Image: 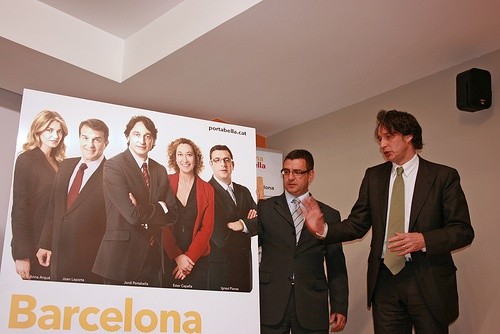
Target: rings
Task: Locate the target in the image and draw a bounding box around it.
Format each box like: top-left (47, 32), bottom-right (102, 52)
top-left (182, 269), bottom-right (187, 274)
top-left (403, 245), bottom-right (406, 249)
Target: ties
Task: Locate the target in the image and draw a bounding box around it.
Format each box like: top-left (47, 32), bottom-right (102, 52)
top-left (291, 198), bottom-right (305, 245)
top-left (65, 163), bottom-right (88, 213)
top-left (227, 185), bottom-right (237, 207)
top-left (142, 163), bottom-right (150, 192)
top-left (383, 167), bottom-right (406, 276)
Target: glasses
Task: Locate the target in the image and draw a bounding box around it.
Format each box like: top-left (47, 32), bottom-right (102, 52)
top-left (213, 158), bottom-right (232, 164)
top-left (281, 169), bottom-right (308, 176)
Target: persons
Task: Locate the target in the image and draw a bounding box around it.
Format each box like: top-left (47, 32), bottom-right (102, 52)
top-left (36, 117), bottom-right (108, 286)
top-left (298, 110), bottom-right (475, 334)
top-left (257, 149), bottom-right (349, 334)
top-left (207, 145), bottom-right (257, 292)
top-left (92, 117), bottom-right (179, 286)
top-left (11, 111), bottom-right (68, 281)
top-left (162, 138), bottom-right (215, 290)
